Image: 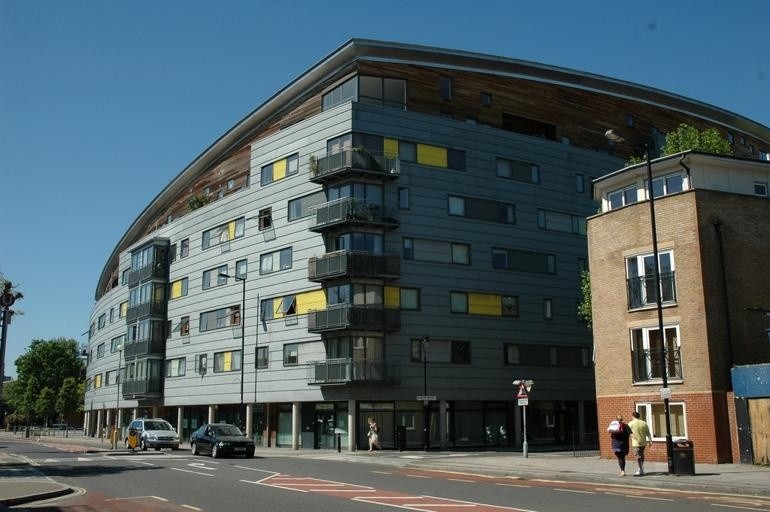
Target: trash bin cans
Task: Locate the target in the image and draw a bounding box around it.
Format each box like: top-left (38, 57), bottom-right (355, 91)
top-left (672, 439), bottom-right (695, 476)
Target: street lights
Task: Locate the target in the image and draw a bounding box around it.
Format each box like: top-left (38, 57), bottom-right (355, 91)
top-left (421, 334), bottom-right (430, 452)
top-left (604, 129), bottom-right (675, 474)
top-left (117, 345), bottom-right (124, 429)
top-left (218, 272), bottom-right (245, 433)
top-left (512, 380), bottom-right (534, 458)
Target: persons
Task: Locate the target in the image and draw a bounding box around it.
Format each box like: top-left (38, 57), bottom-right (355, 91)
top-left (607, 415), bottom-right (641, 477)
top-left (367, 416), bottom-right (382, 452)
top-left (628, 412), bottom-right (654, 476)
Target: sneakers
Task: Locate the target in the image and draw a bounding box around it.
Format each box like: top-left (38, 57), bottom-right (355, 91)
top-left (620, 469), bottom-right (644, 476)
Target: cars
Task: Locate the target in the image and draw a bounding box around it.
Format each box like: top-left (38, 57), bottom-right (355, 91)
top-left (125, 418), bottom-right (180, 451)
top-left (190, 423), bottom-right (255, 458)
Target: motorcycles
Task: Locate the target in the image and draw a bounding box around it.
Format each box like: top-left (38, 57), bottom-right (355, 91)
top-left (480, 424), bottom-right (509, 444)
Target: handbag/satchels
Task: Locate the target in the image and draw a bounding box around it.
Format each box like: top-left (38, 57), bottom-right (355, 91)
top-left (607, 421), bottom-right (624, 433)
